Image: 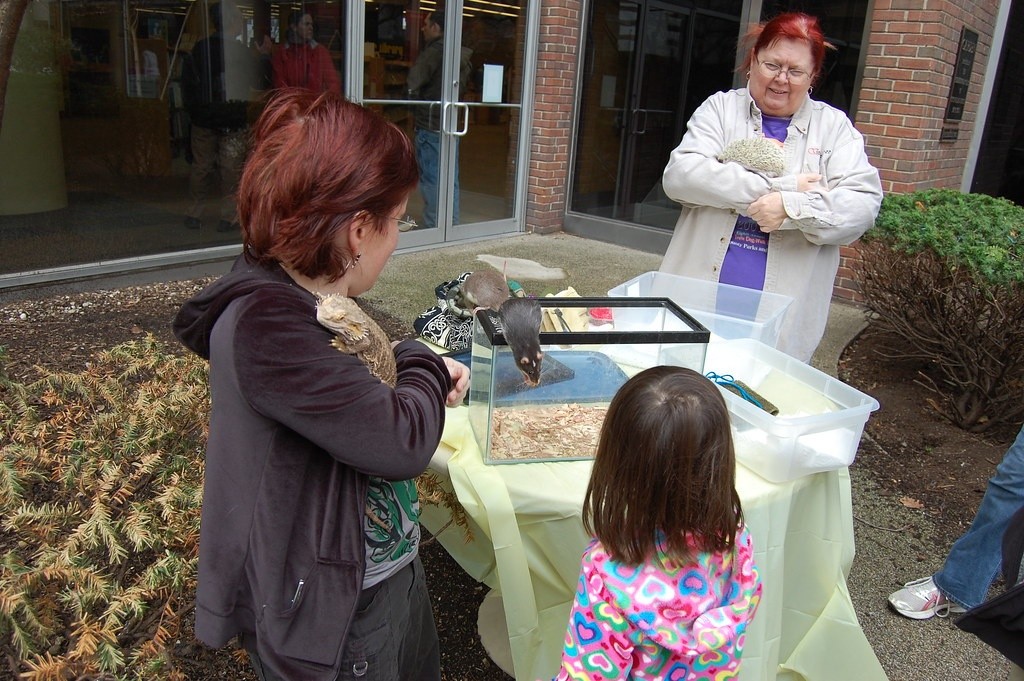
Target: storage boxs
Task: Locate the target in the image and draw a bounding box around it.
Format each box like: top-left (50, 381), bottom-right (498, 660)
top-left (468, 297), bottom-right (711, 466)
top-left (655, 339), bottom-right (880, 482)
top-left (607, 268), bottom-right (796, 349)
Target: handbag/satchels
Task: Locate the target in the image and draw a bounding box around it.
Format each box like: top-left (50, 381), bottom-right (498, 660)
top-left (539, 285), bottom-right (591, 350)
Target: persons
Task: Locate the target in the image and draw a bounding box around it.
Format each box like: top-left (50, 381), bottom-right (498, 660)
top-left (885, 415), bottom-right (1024, 621)
top-left (406, 8), bottom-right (472, 229)
top-left (547, 365), bottom-right (763, 681)
top-left (191, 95), bottom-right (473, 680)
top-left (662, 9), bottom-right (884, 367)
top-left (185, 1), bottom-right (276, 234)
top-left (267, 8), bottom-right (341, 99)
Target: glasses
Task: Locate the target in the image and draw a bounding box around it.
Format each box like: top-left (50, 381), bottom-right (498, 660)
top-left (755, 52), bottom-right (817, 86)
top-left (384, 214), bottom-right (418, 232)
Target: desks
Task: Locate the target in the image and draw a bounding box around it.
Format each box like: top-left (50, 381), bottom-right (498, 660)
top-left (413, 331), bottom-right (889, 681)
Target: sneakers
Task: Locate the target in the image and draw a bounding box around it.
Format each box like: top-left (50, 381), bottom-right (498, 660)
top-left (888, 576), bottom-right (968, 619)
